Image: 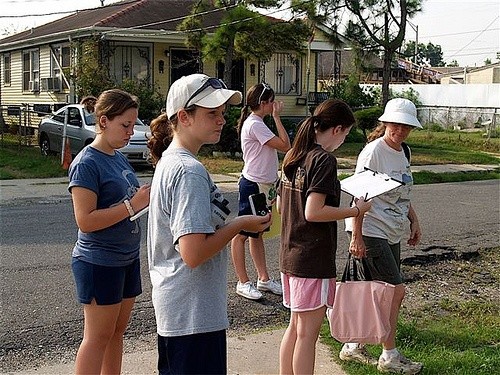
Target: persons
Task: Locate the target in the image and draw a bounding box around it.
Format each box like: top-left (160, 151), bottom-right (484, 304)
top-left (67, 89), bottom-right (150, 375)
top-left (276, 99), bottom-right (374, 375)
top-left (236, 83), bottom-right (290, 301)
top-left (146, 74), bottom-right (274, 375)
top-left (339, 98), bottom-right (423, 375)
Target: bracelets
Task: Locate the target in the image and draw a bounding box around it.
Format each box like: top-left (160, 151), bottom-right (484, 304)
top-left (355, 206), bottom-right (360, 217)
top-left (124, 200), bottom-right (134, 217)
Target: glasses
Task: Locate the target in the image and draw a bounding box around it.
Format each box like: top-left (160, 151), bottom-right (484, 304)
top-left (258, 83), bottom-right (271, 104)
top-left (184, 78), bottom-right (228, 109)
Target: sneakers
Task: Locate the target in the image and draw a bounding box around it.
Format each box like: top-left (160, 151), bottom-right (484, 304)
top-left (376, 353), bottom-right (424, 375)
top-left (339, 342), bottom-right (378, 365)
top-left (257, 275), bottom-right (283, 294)
top-left (236, 280), bottom-right (263, 299)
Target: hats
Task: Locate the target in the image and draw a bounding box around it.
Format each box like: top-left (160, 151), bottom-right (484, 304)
top-left (378, 98), bottom-right (423, 129)
top-left (166, 74), bottom-right (242, 121)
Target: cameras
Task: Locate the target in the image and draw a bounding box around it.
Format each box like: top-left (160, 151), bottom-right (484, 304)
top-left (248, 193), bottom-right (268, 216)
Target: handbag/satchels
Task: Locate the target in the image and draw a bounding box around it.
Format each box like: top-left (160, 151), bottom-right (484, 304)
top-left (325, 252), bottom-right (396, 344)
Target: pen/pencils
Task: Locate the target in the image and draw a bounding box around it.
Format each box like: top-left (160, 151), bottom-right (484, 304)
top-left (363, 192), bottom-right (368, 203)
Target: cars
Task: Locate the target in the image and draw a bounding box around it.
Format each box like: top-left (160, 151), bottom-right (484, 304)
top-left (37, 104), bottom-right (156, 164)
